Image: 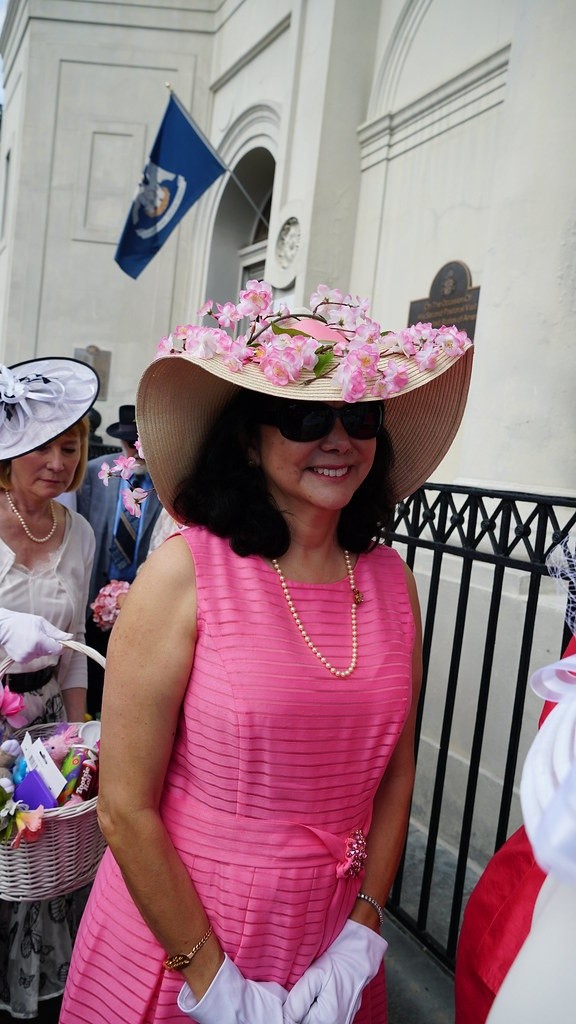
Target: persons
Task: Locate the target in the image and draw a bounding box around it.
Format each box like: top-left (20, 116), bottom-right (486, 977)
top-left (0, 357), bottom-right (185, 1024)
top-left (60, 281), bottom-right (474, 1024)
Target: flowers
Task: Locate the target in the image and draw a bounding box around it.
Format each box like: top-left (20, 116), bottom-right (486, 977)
top-left (97, 440), bottom-right (156, 519)
top-left (0, 683), bottom-right (29, 729)
top-left (0, 785), bottom-right (46, 849)
top-left (157, 274), bottom-right (470, 403)
top-left (90, 579), bottom-right (130, 632)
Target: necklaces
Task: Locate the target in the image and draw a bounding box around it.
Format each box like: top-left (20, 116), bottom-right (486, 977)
top-left (6, 489), bottom-right (58, 543)
top-left (273, 550), bottom-right (363, 678)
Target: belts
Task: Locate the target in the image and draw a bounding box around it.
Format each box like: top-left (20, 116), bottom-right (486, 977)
top-left (2, 666), bottom-right (53, 693)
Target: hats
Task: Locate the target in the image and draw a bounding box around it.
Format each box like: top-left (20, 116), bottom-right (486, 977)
top-left (106, 405), bottom-right (138, 440)
top-left (137, 280), bottom-right (475, 527)
top-left (0, 357), bottom-right (100, 462)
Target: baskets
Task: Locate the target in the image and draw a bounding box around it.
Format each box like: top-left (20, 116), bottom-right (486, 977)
top-left (0, 640), bottom-right (109, 903)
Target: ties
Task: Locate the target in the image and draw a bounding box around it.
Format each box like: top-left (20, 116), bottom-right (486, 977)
top-left (112, 474), bottom-right (144, 571)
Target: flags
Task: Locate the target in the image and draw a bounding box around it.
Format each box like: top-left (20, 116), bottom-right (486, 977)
top-left (115, 96), bottom-right (224, 278)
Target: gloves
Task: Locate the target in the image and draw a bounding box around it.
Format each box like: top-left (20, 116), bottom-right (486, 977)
top-left (0, 607), bottom-right (74, 663)
top-left (177, 952), bottom-right (289, 1024)
top-left (282, 918), bottom-right (388, 1024)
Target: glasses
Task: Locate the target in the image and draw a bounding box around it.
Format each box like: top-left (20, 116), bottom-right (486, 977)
top-left (256, 400), bottom-right (385, 441)
top-left (127, 440), bottom-right (136, 449)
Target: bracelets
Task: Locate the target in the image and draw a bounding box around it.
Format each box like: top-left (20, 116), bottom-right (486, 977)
top-left (162, 927), bottom-right (212, 971)
top-left (358, 893), bottom-right (383, 926)
